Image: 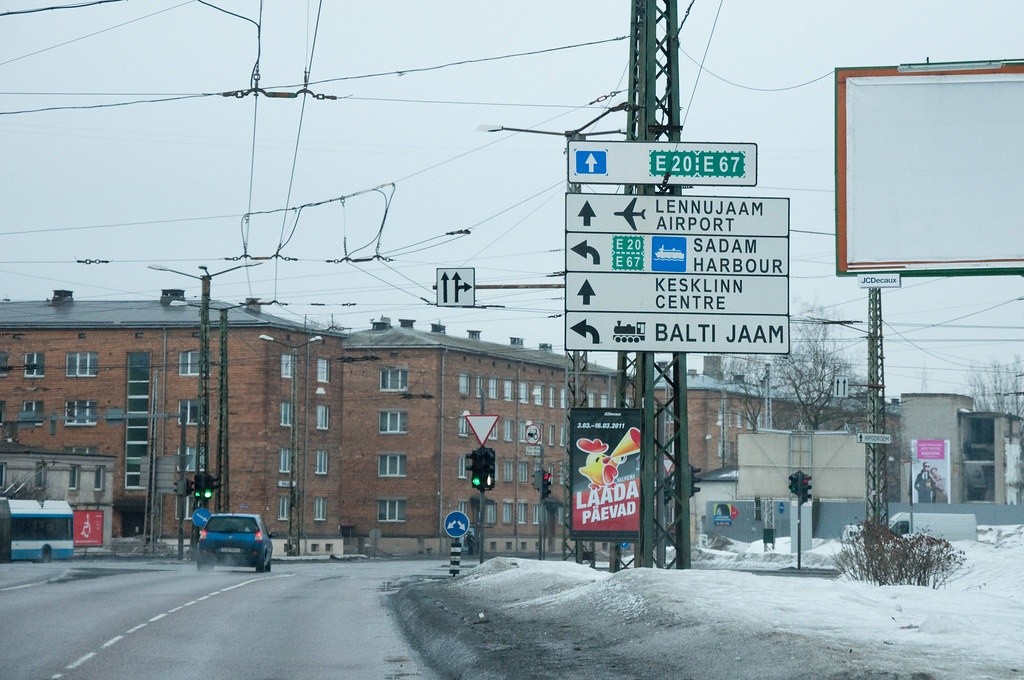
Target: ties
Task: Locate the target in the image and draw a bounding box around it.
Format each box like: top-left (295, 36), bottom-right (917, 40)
top-left (924, 472), bottom-right (927, 480)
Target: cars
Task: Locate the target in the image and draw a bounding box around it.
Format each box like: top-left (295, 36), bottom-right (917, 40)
top-left (197, 512), bottom-right (279, 573)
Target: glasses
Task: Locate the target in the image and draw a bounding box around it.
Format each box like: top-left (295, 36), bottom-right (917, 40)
top-left (925, 465), bottom-right (930, 468)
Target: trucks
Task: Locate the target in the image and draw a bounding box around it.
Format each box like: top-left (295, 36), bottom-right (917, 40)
top-left (887, 511), bottom-right (979, 545)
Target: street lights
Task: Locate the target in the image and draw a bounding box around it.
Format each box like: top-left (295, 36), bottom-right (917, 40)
top-left (144, 260), bottom-right (265, 565)
top-left (258, 333), bottom-right (324, 557)
top-left (168, 299), bottom-right (286, 513)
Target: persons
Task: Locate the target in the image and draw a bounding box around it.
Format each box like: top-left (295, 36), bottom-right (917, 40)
top-left (930, 467), bottom-right (948, 503)
top-left (467, 531), bottom-right (475, 554)
top-left (914, 462), bottom-right (936, 503)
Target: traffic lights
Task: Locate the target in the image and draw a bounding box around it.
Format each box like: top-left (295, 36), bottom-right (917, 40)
top-left (193, 473), bottom-right (204, 499)
top-left (689, 464), bottom-right (701, 498)
top-left (531, 470), bottom-right (540, 491)
top-left (482, 450), bottom-right (495, 488)
top-left (465, 450), bottom-right (475, 471)
top-left (788, 473), bottom-right (800, 494)
top-left (204, 475), bottom-right (215, 499)
top-left (801, 473), bottom-right (813, 503)
top-left (185, 477), bottom-right (195, 496)
top-left (540, 471), bottom-right (552, 500)
top-left (471, 450), bottom-right (483, 490)
top-left (663, 476), bottom-right (672, 506)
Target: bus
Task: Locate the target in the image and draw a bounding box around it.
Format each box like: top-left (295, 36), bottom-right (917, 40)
top-left (0, 461), bottom-right (75, 564)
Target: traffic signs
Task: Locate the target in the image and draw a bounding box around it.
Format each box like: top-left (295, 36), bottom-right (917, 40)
top-left (566, 140), bottom-right (758, 187)
top-left (565, 192), bottom-right (790, 354)
top-left (855, 432), bottom-right (892, 445)
